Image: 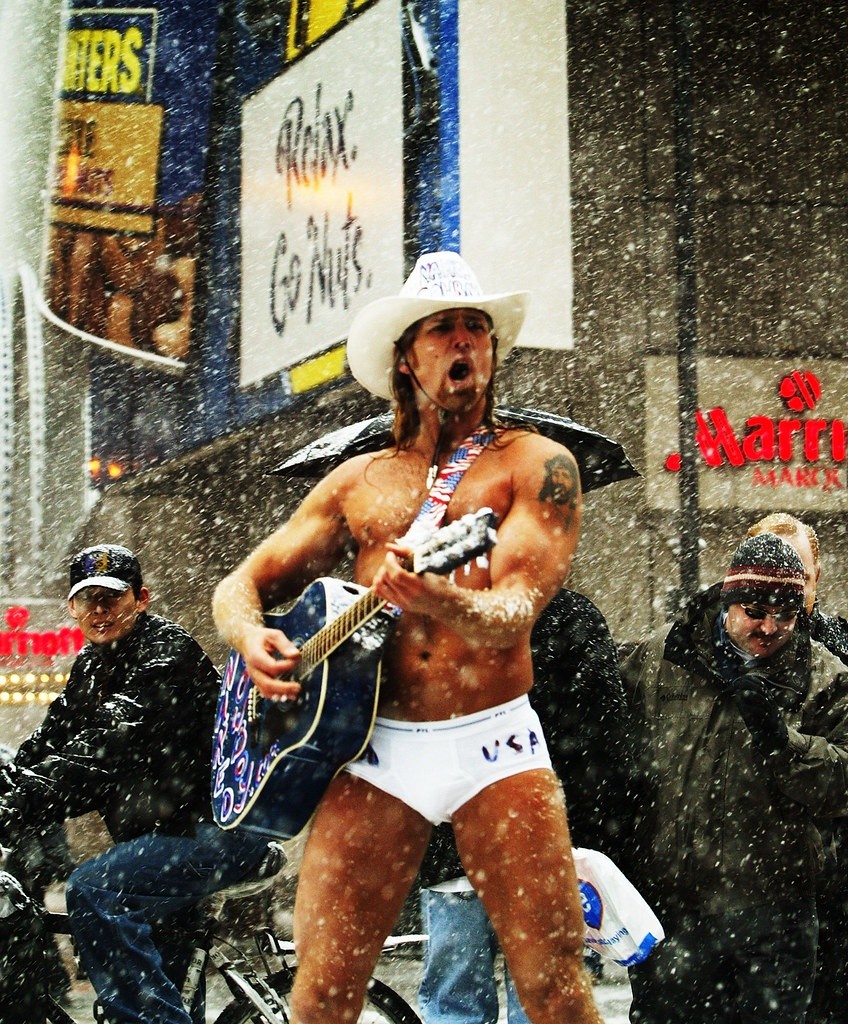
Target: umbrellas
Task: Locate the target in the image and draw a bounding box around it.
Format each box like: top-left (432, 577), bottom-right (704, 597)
top-left (265, 400), bottom-right (640, 494)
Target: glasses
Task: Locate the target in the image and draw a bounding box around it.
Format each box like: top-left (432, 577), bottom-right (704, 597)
top-left (742, 603), bottom-right (799, 622)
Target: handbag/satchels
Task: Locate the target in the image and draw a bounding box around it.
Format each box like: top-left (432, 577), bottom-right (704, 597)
top-left (571, 847), bottom-right (665, 967)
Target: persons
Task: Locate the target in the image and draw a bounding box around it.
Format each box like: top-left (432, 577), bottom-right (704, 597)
top-left (616, 532), bottom-right (848, 1024)
top-left (212, 249), bottom-right (608, 1024)
top-left (417, 588), bottom-right (626, 1024)
top-left (1, 543), bottom-right (288, 1024)
top-left (746, 512), bottom-right (848, 665)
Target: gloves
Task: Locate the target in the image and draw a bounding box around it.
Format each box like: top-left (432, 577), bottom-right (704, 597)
top-left (723, 675), bottom-right (788, 755)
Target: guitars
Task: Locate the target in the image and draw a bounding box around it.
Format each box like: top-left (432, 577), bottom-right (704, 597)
top-left (210, 507), bottom-right (499, 846)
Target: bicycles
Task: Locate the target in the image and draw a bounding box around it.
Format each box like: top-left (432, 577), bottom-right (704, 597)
top-left (0, 757), bottom-right (421, 1023)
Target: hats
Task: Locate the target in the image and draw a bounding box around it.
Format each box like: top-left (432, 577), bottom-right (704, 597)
top-left (719, 532), bottom-right (805, 609)
top-left (348, 251), bottom-right (530, 400)
top-left (67, 544), bottom-right (140, 601)
